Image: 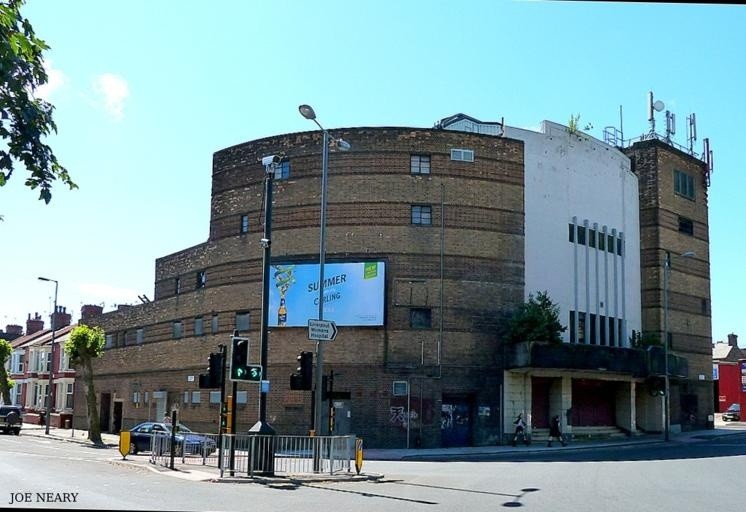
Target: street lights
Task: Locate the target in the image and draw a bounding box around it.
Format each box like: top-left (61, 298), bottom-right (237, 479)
top-left (36, 277), bottom-right (59, 435)
top-left (662, 250), bottom-right (697, 441)
top-left (297, 103), bottom-right (352, 473)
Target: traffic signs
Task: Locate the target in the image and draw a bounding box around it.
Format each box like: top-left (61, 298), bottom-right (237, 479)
top-left (308, 318), bottom-right (338, 342)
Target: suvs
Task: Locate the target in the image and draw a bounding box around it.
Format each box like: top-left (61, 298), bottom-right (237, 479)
top-left (0, 404), bottom-right (24, 436)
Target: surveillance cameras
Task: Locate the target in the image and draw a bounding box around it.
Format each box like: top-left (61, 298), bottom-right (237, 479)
top-left (261, 154), bottom-right (282, 168)
top-left (261, 238), bottom-right (272, 248)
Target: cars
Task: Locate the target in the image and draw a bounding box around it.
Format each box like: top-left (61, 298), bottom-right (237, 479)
top-left (127, 422), bottom-right (217, 457)
top-left (721, 403), bottom-right (740, 421)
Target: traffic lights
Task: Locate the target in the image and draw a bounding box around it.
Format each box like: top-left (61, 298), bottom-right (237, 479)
top-left (330, 404), bottom-right (335, 432)
top-left (229, 337), bottom-right (263, 383)
top-left (288, 350), bottom-right (313, 392)
top-left (198, 351), bottom-right (224, 389)
top-left (221, 402), bottom-right (228, 429)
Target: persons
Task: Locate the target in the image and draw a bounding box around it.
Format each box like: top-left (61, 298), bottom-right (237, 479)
top-left (547, 415), bottom-right (568, 448)
top-left (162, 411), bottom-right (172, 423)
top-left (39, 408), bottom-right (47, 427)
top-left (511, 412), bottom-right (530, 447)
top-left (688, 412), bottom-right (696, 431)
